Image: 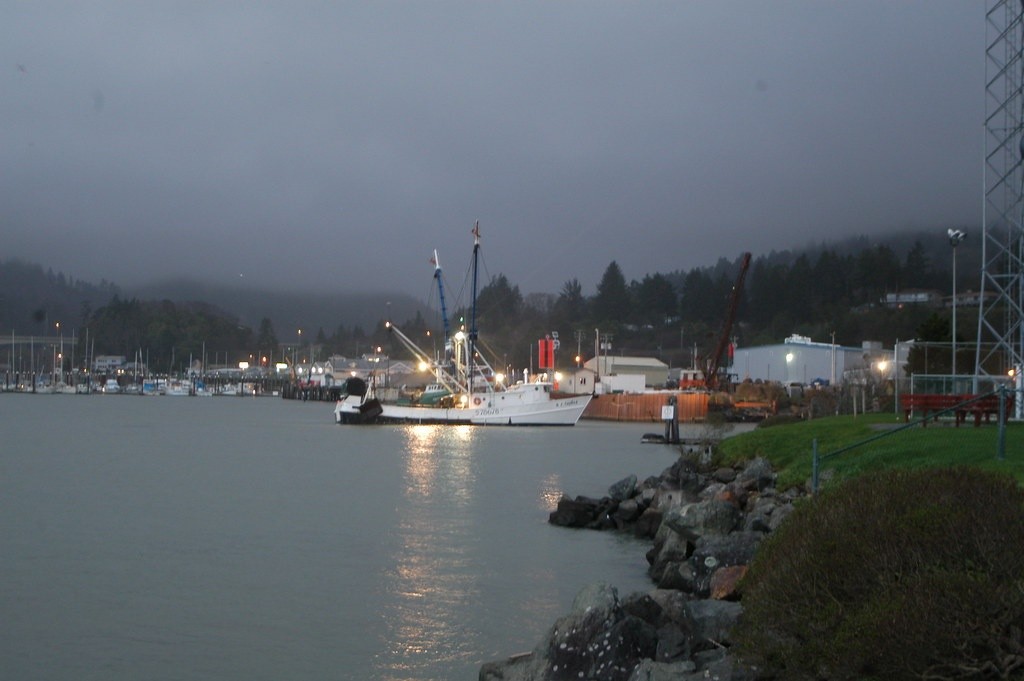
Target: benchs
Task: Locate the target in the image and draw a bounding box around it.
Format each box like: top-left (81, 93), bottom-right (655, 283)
top-left (898, 392), bottom-right (1013, 428)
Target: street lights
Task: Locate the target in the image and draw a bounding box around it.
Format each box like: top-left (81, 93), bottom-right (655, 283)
top-left (945, 229), bottom-right (966, 397)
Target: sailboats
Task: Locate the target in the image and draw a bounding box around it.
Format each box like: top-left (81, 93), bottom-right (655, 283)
top-left (335, 218), bottom-right (595, 426)
top-left (0, 329), bottom-right (259, 397)
